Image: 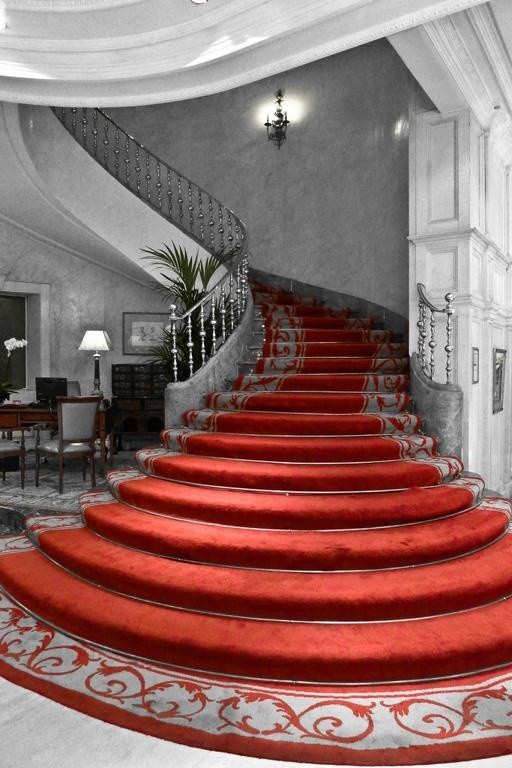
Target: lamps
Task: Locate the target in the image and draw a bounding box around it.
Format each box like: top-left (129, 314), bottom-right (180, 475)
top-left (76, 330), bottom-right (116, 396)
top-left (262, 85), bottom-right (292, 150)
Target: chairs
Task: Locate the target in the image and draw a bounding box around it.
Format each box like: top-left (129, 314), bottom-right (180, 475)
top-left (1, 426), bottom-right (32, 490)
top-left (34, 394), bottom-right (105, 495)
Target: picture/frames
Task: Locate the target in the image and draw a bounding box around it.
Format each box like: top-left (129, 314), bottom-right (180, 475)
top-left (121, 311), bottom-right (173, 357)
top-left (470, 346), bottom-right (479, 385)
top-left (491, 346), bottom-right (507, 417)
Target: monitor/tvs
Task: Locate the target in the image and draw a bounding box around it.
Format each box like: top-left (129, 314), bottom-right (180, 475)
top-left (36, 378), bottom-right (67, 399)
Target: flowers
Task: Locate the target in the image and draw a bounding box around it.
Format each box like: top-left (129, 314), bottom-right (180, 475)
top-left (1, 336), bottom-right (29, 405)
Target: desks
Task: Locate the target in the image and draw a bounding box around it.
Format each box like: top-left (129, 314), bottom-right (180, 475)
top-left (1, 402), bottom-right (111, 480)
top-left (109, 394), bottom-right (165, 455)
